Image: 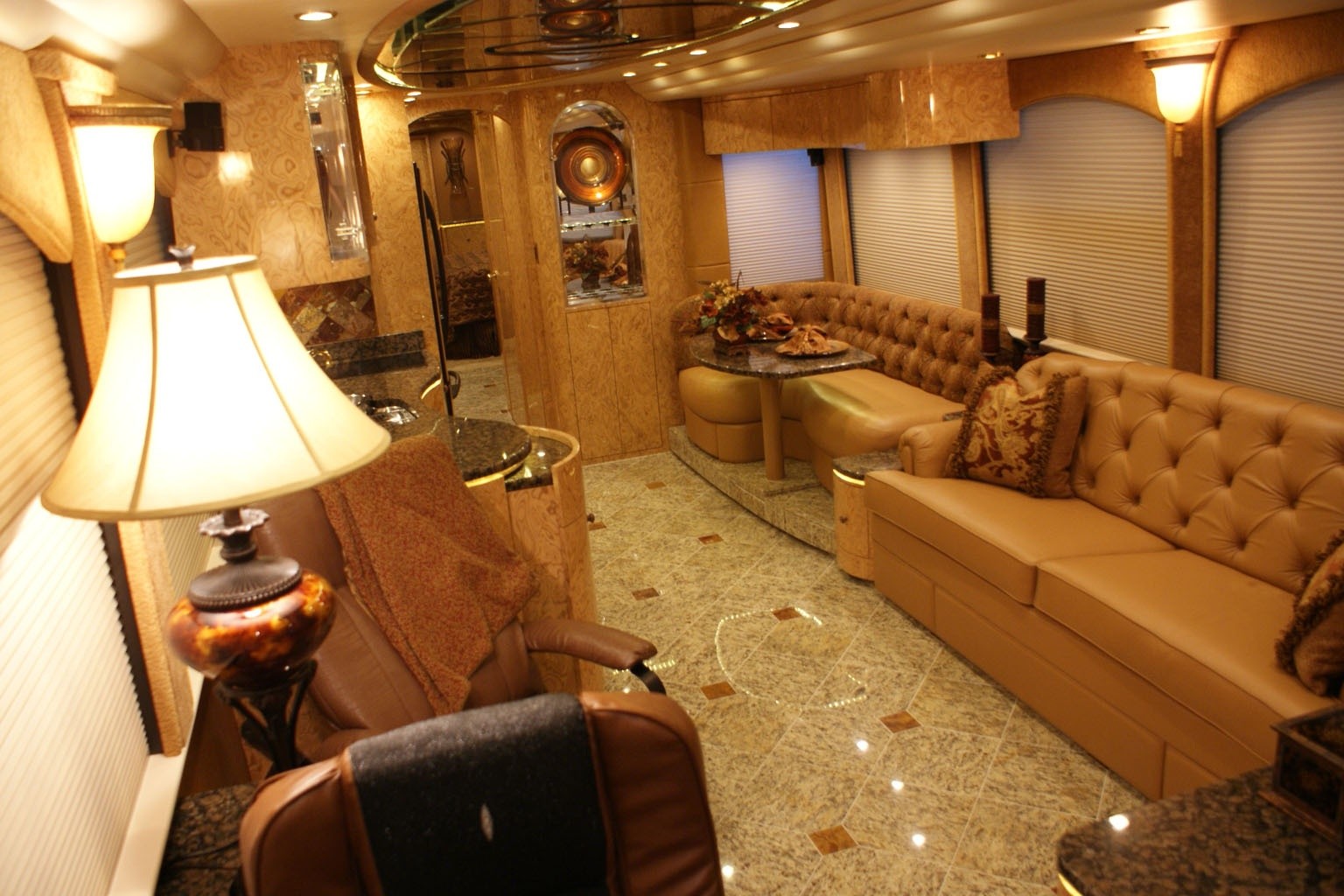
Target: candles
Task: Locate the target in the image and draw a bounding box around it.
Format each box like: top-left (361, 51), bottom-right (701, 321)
top-left (981, 293), bottom-right (998, 353)
top-left (1027, 278), bottom-right (1047, 338)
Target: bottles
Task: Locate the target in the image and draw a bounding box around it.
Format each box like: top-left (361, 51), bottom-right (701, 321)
top-left (626, 223), bottom-right (642, 286)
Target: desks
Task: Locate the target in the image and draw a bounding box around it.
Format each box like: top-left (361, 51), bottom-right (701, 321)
top-left (690, 329), bottom-right (876, 493)
top-left (157, 783), bottom-right (258, 896)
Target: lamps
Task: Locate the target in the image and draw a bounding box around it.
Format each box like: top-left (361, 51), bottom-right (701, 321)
top-left (1151, 62), bottom-right (1211, 157)
top-left (64, 52), bottom-right (392, 778)
top-left (440, 136), bottom-right (472, 195)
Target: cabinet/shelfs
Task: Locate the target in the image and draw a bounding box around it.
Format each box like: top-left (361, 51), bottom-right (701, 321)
top-left (549, 99), bottom-right (647, 304)
top-left (467, 424), bottom-right (604, 695)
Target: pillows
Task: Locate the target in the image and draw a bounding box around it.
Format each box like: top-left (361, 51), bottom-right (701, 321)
top-left (943, 360), bottom-right (1091, 499)
top-left (1274, 529), bottom-right (1344, 698)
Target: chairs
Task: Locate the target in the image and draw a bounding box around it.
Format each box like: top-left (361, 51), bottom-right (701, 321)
top-left (237, 435), bottom-right (725, 896)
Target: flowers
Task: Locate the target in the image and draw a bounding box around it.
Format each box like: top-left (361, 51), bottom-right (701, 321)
top-left (678, 269), bottom-right (772, 343)
top-left (563, 235), bottom-right (610, 279)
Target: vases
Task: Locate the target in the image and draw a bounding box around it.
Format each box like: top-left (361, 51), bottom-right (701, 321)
top-left (581, 271), bottom-right (602, 290)
top-left (711, 322), bottom-right (750, 358)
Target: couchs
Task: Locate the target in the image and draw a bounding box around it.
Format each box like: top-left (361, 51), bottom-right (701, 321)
top-left (442, 270), bottom-right (498, 357)
top-left (600, 237), bottom-right (628, 286)
top-left (670, 282), bottom-right (1344, 801)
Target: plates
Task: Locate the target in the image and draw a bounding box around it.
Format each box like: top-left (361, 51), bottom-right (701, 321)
top-left (775, 339), bottom-right (849, 357)
top-left (553, 125), bottom-right (629, 206)
